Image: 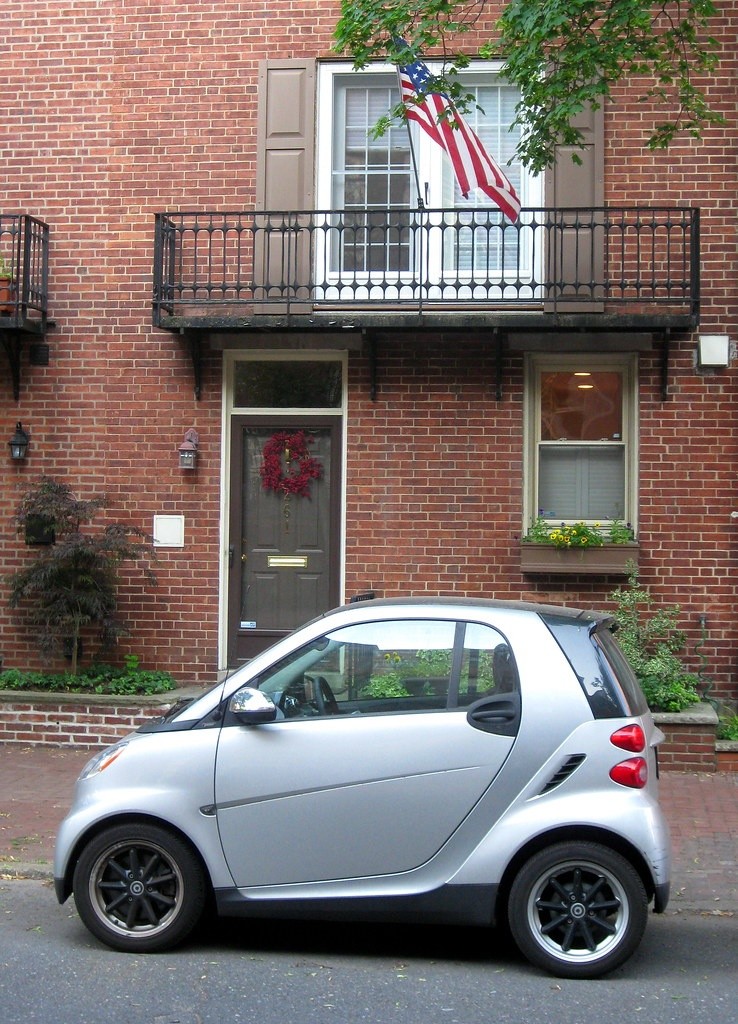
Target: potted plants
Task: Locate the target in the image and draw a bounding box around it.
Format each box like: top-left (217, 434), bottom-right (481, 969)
top-left (0, 253), bottom-right (17, 313)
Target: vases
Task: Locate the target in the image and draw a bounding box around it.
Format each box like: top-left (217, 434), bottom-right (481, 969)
top-left (402, 676), bottom-right (449, 696)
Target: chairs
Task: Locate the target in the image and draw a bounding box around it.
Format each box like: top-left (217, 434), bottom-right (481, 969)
top-left (480, 643), bottom-right (513, 697)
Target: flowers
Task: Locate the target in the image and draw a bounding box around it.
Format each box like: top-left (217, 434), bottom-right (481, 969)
top-left (259, 429), bottom-right (324, 498)
top-left (512, 508), bottom-right (635, 551)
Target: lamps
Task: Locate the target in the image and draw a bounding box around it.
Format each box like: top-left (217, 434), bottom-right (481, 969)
top-left (7, 421), bottom-right (31, 460)
top-left (176, 427), bottom-right (199, 470)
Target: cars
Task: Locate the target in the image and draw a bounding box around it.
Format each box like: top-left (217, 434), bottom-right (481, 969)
top-left (52, 588), bottom-right (673, 981)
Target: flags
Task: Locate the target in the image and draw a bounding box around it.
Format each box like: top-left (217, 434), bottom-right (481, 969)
top-left (391, 26), bottom-right (523, 226)
